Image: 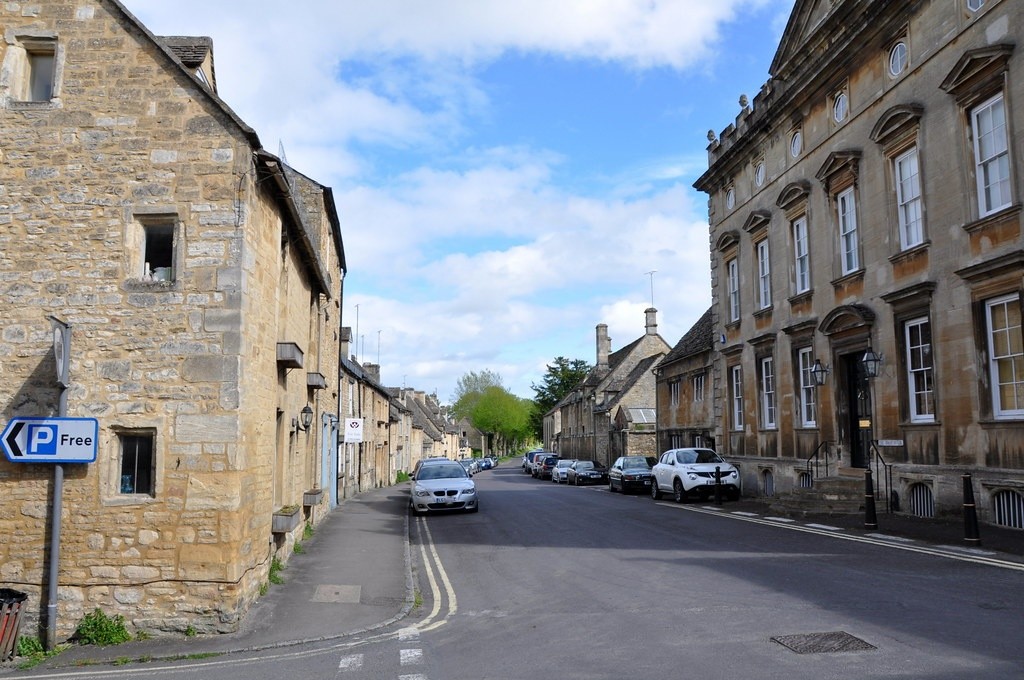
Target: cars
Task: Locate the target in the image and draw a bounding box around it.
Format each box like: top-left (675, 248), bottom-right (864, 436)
top-left (460, 455), bottom-right (498, 478)
top-left (551, 459), bottom-right (579, 484)
top-left (607, 456), bottom-right (659, 495)
top-left (522, 448), bottom-right (545, 474)
top-left (652, 447), bottom-right (740, 503)
top-left (408, 457), bottom-right (450, 477)
top-left (567, 460), bottom-right (609, 486)
top-left (537, 457), bottom-right (563, 481)
top-left (409, 459), bottom-right (479, 516)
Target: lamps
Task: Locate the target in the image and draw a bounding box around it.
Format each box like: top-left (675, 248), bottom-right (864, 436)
top-left (810, 358), bottom-right (830, 386)
top-left (862, 347), bottom-right (883, 377)
top-left (295, 403), bottom-right (314, 434)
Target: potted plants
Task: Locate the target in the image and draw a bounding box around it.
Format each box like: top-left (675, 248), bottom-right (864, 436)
top-left (304, 489), bottom-right (323, 506)
top-left (274, 504), bottom-right (300, 534)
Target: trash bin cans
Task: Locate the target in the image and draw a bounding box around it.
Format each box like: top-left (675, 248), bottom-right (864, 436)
top-left (511, 451), bottom-right (515, 455)
top-left (0, 588), bottom-right (28, 662)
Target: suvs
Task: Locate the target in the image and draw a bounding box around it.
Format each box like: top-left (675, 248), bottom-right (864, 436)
top-left (530, 452), bottom-right (558, 479)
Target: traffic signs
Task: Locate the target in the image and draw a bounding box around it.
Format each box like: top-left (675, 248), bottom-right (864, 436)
top-left (0, 417), bottom-right (98, 464)
